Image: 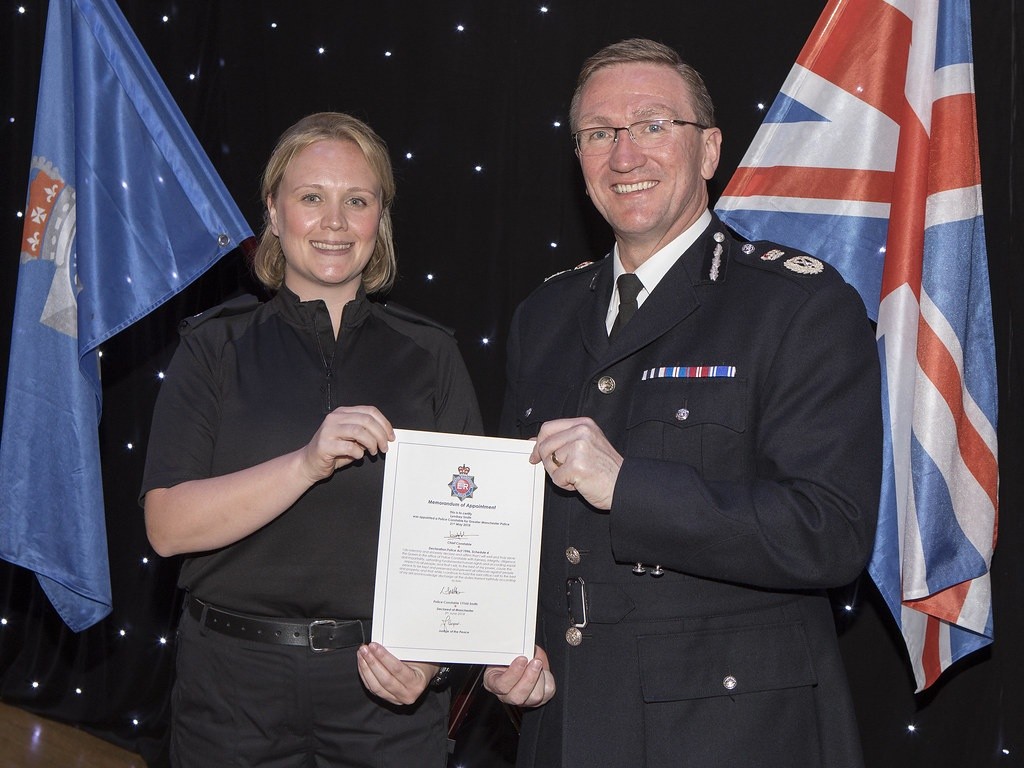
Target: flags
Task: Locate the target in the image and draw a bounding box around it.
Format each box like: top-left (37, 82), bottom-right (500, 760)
top-left (0, 0), bottom-right (254, 635)
top-left (712, 0), bottom-right (1002, 699)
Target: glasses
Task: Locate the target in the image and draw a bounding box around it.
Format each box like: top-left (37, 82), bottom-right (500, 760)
top-left (568, 119), bottom-right (710, 156)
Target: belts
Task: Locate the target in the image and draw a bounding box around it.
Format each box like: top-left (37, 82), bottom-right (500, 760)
top-left (189, 599), bottom-right (373, 654)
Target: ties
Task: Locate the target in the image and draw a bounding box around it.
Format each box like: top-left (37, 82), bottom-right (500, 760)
top-left (609, 272), bottom-right (644, 346)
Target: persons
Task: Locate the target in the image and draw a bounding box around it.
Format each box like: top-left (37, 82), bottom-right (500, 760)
top-left (483, 35), bottom-right (887, 768)
top-left (139, 118), bottom-right (484, 768)
